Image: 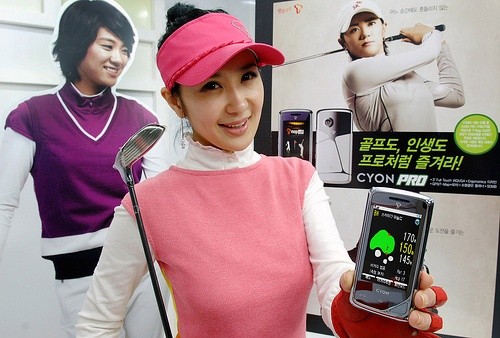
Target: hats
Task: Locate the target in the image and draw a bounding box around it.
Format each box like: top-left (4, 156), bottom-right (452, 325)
top-left (338, 0), bottom-right (384, 38)
top-left (156, 13), bottom-right (285, 90)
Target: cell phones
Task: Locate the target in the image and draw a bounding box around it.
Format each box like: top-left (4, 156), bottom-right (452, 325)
top-left (315, 108), bottom-right (353, 184)
top-left (349, 187), bottom-right (434, 323)
top-left (279, 108), bottom-right (313, 168)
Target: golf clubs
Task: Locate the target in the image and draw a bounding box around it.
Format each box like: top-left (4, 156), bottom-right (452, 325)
top-left (115, 123), bottom-right (173, 338)
top-left (258, 24), bottom-right (446, 73)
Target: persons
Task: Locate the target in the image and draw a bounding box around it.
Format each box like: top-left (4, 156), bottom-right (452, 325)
top-left (337, 1), bottom-right (465, 132)
top-left (74, 3), bottom-right (448, 338)
top-left (0, 0), bottom-right (169, 338)
top-left (284, 127), bottom-right (306, 159)
